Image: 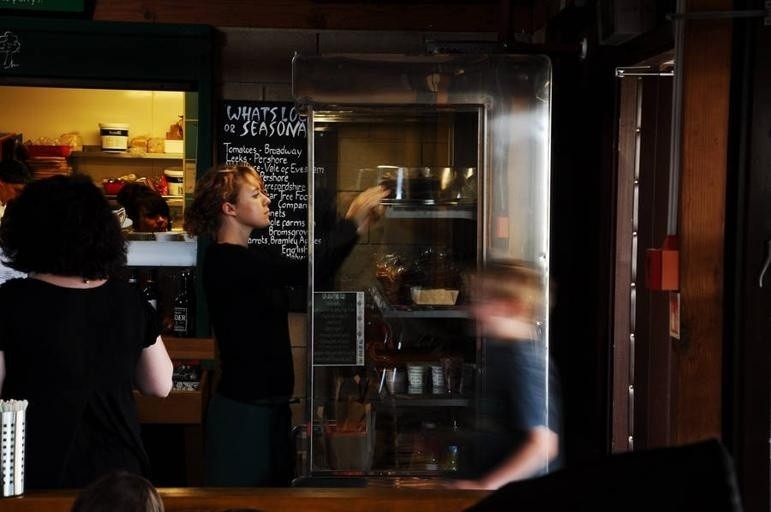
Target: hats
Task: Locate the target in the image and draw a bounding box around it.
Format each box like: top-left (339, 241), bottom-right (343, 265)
top-left (0, 159), bottom-right (31, 184)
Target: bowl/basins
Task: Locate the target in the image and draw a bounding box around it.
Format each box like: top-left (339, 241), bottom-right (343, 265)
top-left (23, 144), bottom-right (74, 156)
top-left (323, 431), bottom-right (377, 478)
top-left (102, 182), bottom-right (124, 194)
top-left (154, 231), bottom-right (178, 242)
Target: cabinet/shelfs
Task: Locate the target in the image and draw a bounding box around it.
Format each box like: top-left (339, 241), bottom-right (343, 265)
top-left (131, 336), bottom-right (216, 423)
top-left (0, 88), bottom-right (184, 238)
top-left (314, 102), bottom-right (500, 489)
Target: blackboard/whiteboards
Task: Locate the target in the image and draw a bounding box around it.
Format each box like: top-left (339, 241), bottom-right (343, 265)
top-left (314, 291), bottom-right (365, 367)
top-left (214, 99), bottom-right (337, 313)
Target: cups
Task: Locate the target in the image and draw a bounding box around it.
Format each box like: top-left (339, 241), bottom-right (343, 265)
top-left (375, 163), bottom-right (477, 207)
top-left (385, 368), bottom-right (406, 396)
top-left (406, 365), bottom-right (425, 396)
top-left (432, 365), bottom-right (447, 395)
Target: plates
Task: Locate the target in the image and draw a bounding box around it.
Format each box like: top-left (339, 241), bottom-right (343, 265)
top-left (29, 155), bottom-right (70, 181)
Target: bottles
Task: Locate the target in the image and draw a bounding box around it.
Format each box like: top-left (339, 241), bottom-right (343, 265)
top-left (410, 422), bottom-right (446, 480)
top-left (444, 425), bottom-right (460, 472)
top-left (142, 277), bottom-right (160, 313)
top-left (128, 269), bottom-right (138, 285)
top-left (171, 268), bottom-right (193, 338)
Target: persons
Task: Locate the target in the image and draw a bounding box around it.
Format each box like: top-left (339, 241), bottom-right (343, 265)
top-left (448, 259), bottom-right (563, 509)
top-left (0, 157), bottom-right (173, 511)
top-left (184, 165), bottom-right (392, 487)
